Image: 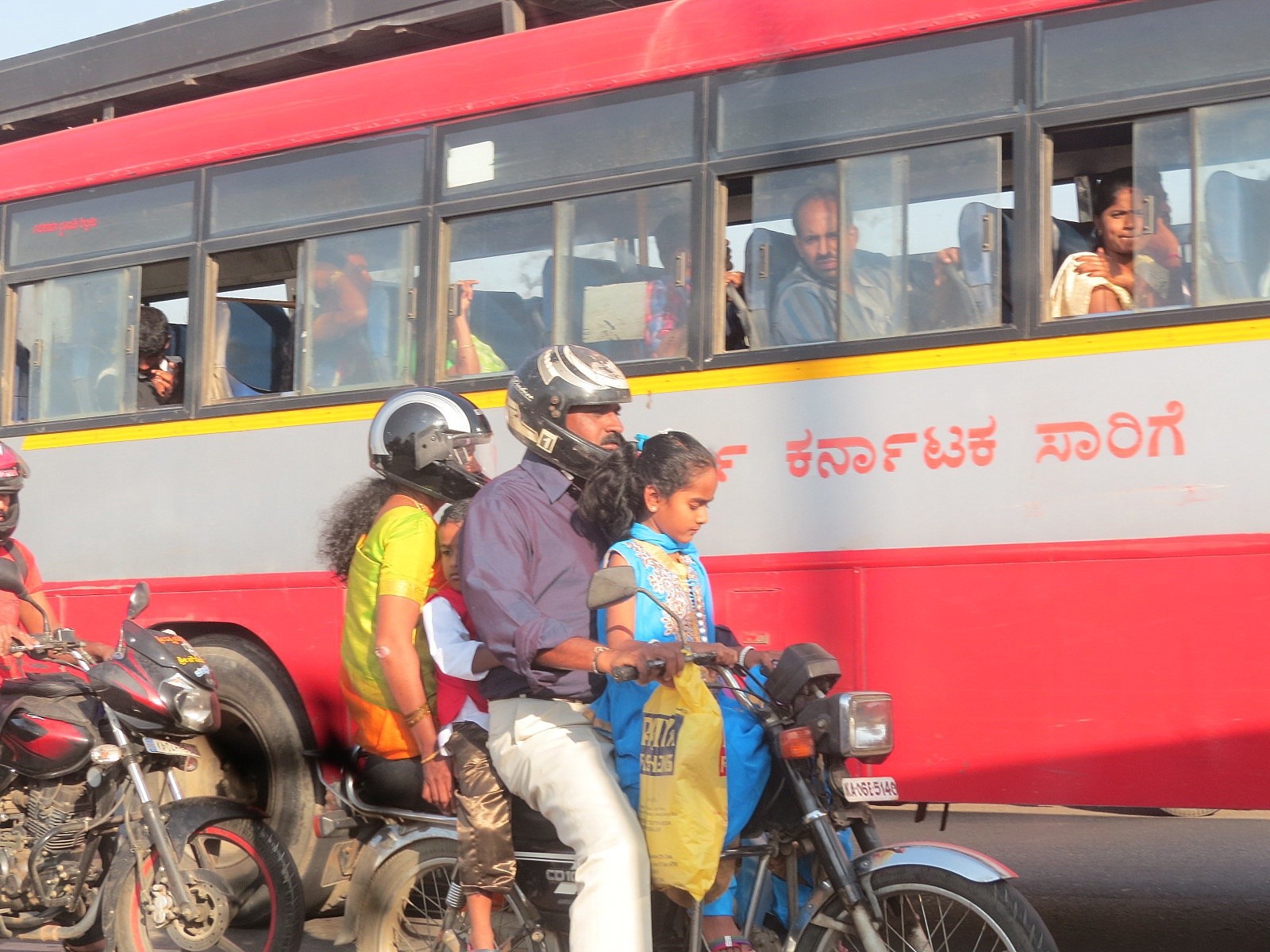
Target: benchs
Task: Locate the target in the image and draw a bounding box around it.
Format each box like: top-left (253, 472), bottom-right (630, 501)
top-left (0, 171), bottom-right (1270, 421)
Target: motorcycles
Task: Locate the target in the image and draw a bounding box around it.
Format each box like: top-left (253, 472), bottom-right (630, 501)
top-left (314, 563), bottom-right (1056, 952)
top-left (0, 554), bottom-right (304, 952)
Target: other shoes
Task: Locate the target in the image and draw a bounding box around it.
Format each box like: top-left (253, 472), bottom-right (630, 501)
top-left (466, 929), bottom-right (499, 952)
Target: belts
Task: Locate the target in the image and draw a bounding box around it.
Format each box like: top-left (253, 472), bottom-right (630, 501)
top-left (489, 692), bottom-right (586, 704)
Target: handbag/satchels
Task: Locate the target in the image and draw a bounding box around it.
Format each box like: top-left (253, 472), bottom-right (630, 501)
top-left (641, 665), bottom-right (730, 903)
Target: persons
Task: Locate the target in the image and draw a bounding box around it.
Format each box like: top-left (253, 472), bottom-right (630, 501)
top-left (593, 428), bottom-right (853, 952)
top-left (340, 386), bottom-right (493, 810)
top-left (1048, 166), bottom-right (1192, 319)
top-left (278, 244), bottom-right (372, 392)
top-left (634, 209), bottom-right (745, 361)
top-left (772, 189), bottom-right (962, 345)
top-left (394, 275), bottom-right (509, 383)
top-left (0, 440), bottom-right (116, 664)
top-left (94, 306), bottom-right (184, 414)
top-left (462, 344), bottom-right (781, 952)
top-left (421, 497), bottom-right (516, 951)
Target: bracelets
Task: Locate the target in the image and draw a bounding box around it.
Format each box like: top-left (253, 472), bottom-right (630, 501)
top-left (1134, 290), bottom-right (1155, 302)
top-left (738, 646), bottom-right (754, 667)
top-left (405, 704), bottom-right (430, 727)
top-left (420, 750), bottom-right (440, 765)
top-left (592, 645), bottom-right (608, 674)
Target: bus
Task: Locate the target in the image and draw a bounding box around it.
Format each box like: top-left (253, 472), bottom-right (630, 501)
top-left (0, 0), bottom-right (1270, 950)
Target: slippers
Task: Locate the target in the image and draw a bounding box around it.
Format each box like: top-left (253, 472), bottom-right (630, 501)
top-left (697, 915), bottom-right (755, 952)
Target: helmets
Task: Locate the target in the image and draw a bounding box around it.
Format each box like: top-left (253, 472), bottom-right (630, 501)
top-left (368, 388), bottom-right (494, 505)
top-left (508, 342), bottom-right (634, 480)
top-left (0, 440), bottom-right (28, 538)
